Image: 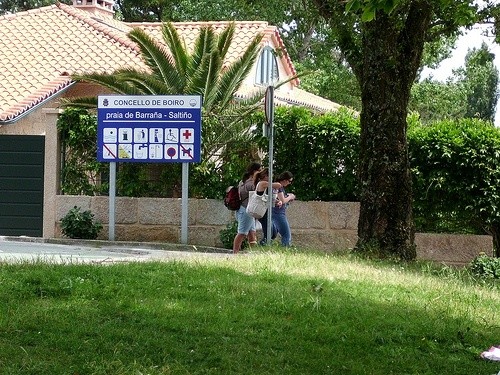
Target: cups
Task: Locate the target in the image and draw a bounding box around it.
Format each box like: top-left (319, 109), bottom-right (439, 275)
top-left (287, 193), bottom-right (293, 203)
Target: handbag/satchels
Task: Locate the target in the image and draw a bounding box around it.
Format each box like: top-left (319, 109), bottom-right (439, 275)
top-left (246, 191), bottom-right (269, 218)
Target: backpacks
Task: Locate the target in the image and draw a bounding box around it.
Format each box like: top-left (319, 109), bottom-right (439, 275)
top-left (225, 180), bottom-right (244, 211)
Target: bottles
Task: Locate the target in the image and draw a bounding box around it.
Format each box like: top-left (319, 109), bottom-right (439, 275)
top-left (273, 188), bottom-right (279, 200)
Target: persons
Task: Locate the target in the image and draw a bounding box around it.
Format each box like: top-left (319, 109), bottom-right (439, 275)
top-left (224, 162), bottom-right (261, 254)
top-left (254, 168), bottom-right (278, 247)
top-left (268, 171), bottom-right (296, 248)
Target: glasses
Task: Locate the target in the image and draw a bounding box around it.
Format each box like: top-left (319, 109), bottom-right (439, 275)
top-left (286, 179), bottom-right (293, 182)
